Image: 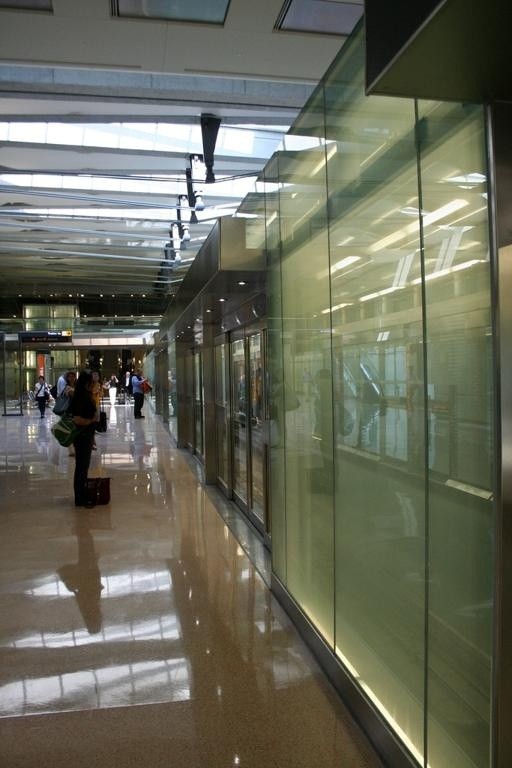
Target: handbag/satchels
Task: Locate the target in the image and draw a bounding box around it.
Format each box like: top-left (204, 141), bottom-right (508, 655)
top-left (44, 389), bottom-right (49, 400)
top-left (53, 396), bottom-right (70, 416)
top-left (143, 382), bottom-right (153, 393)
top-left (49, 385), bottom-right (57, 398)
top-left (88, 477), bottom-right (111, 505)
top-left (95, 412), bottom-right (108, 433)
top-left (50, 413), bottom-right (81, 447)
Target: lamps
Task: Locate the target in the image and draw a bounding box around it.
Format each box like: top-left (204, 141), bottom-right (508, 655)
top-left (171, 112), bottom-right (220, 260)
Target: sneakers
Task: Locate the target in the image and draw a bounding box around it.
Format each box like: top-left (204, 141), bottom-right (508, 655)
top-left (75, 501), bottom-right (95, 509)
top-left (135, 416), bottom-right (145, 419)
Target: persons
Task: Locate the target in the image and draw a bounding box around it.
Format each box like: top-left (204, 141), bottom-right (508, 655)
top-left (32, 367), bottom-right (150, 460)
top-left (67, 370), bottom-right (101, 510)
